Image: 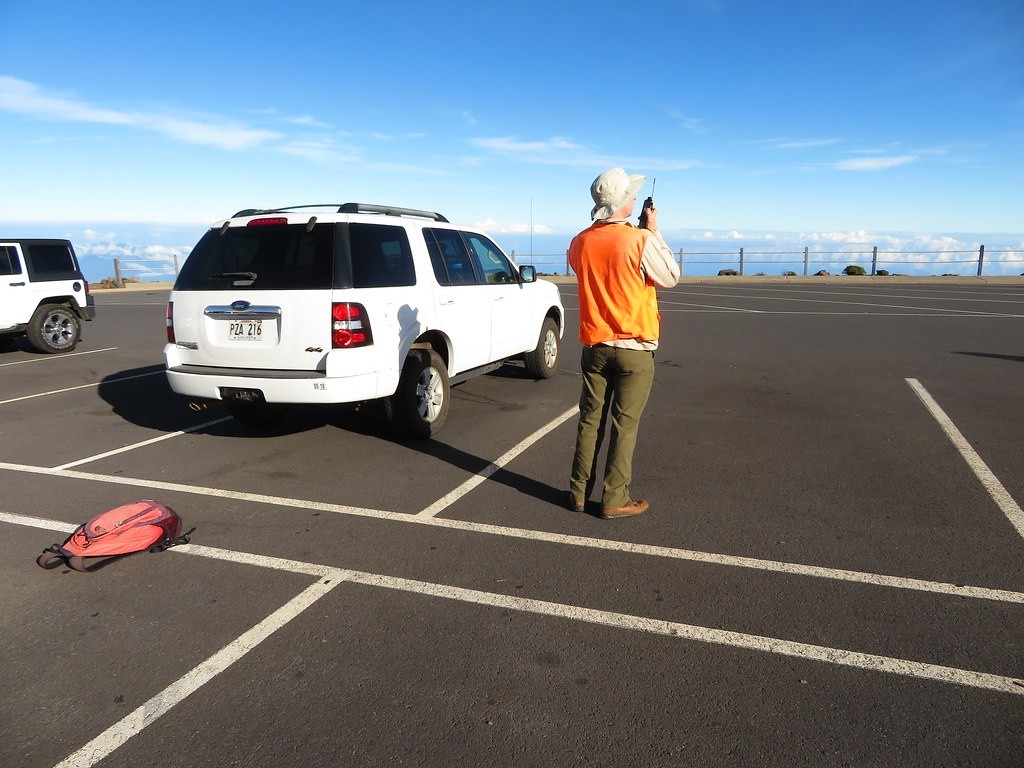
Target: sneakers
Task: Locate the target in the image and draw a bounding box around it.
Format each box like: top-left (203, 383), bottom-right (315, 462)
top-left (600, 498), bottom-right (648, 519)
top-left (570, 493), bottom-right (589, 512)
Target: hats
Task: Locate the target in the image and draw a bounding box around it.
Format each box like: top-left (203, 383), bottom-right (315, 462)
top-left (590, 167), bottom-right (646, 221)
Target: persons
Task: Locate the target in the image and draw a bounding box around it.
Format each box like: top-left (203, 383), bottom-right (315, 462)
top-left (568, 167), bottom-right (680, 518)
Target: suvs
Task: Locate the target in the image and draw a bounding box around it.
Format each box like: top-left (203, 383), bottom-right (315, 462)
top-left (163, 201), bottom-right (565, 440)
top-left (0, 239), bottom-right (96, 354)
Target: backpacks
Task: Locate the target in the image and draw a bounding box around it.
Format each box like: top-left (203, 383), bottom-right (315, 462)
top-left (37, 499), bottom-right (196, 573)
